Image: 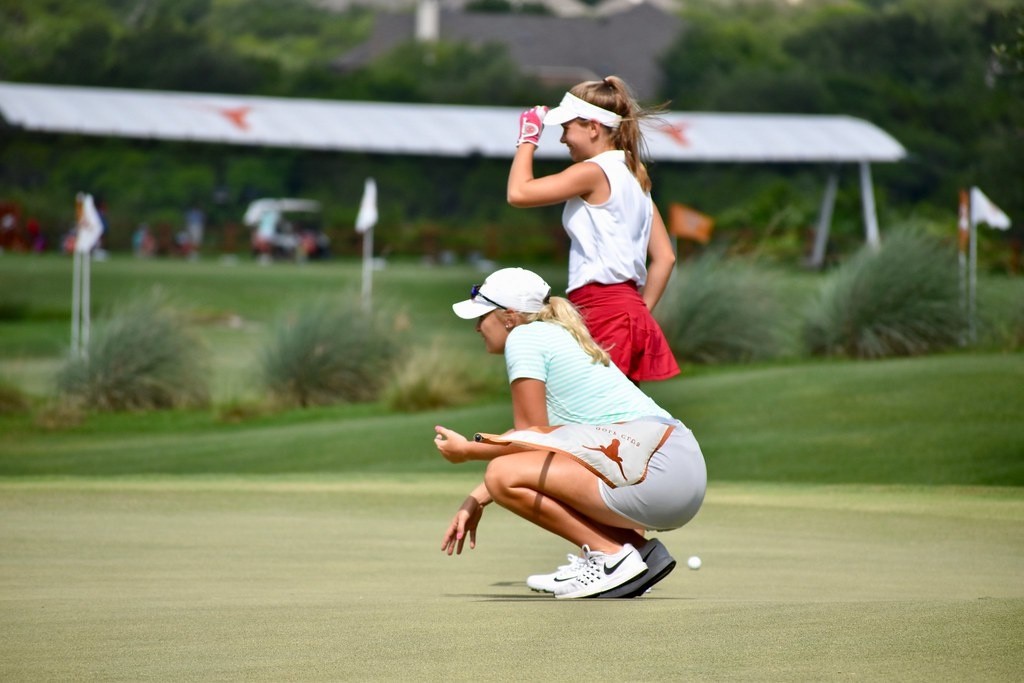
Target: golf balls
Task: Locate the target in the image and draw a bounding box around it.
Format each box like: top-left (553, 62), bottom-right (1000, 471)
top-left (687, 556), bottom-right (702, 570)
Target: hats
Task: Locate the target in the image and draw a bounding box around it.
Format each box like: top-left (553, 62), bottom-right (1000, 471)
top-left (452, 267), bottom-right (552, 319)
top-left (542, 92), bottom-right (623, 128)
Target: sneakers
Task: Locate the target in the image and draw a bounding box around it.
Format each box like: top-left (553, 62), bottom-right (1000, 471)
top-left (553, 544), bottom-right (649, 600)
top-left (526, 553), bottom-right (589, 593)
top-left (598, 538), bottom-right (677, 598)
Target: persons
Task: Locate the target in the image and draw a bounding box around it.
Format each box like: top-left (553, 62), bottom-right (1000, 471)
top-left (250, 206), bottom-right (324, 262)
top-left (435, 267), bottom-right (707, 599)
top-left (131, 203), bottom-right (207, 257)
top-left (0, 199), bottom-right (110, 257)
top-left (506, 76), bottom-right (676, 593)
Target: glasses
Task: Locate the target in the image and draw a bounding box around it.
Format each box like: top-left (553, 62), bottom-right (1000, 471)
top-left (471, 284), bottom-right (508, 311)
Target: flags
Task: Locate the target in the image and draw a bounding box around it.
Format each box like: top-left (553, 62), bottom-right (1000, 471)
top-left (958, 192), bottom-right (968, 254)
top-left (670, 203), bottom-right (711, 243)
top-left (76, 194), bottom-right (102, 251)
top-left (356, 183), bottom-right (377, 232)
top-left (974, 189), bottom-right (1011, 230)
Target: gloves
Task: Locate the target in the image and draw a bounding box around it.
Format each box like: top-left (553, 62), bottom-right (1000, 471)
top-left (516, 105), bottom-right (548, 151)
